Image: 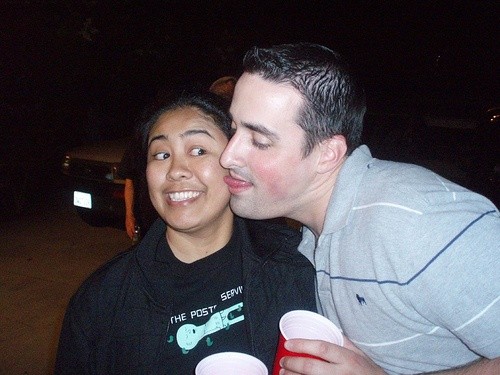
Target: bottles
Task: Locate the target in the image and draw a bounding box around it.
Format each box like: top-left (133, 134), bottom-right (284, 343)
top-left (270, 310), bottom-right (344, 375)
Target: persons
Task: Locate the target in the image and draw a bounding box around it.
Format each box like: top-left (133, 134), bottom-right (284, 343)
top-left (210, 75), bottom-right (238, 107)
top-left (116, 138), bottom-right (158, 243)
top-left (55, 92), bottom-right (319, 375)
top-left (220, 44), bottom-right (500, 375)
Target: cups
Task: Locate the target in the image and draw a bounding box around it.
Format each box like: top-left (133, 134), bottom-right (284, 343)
top-left (195, 352), bottom-right (268, 375)
